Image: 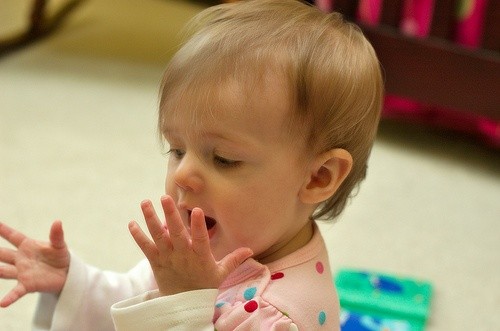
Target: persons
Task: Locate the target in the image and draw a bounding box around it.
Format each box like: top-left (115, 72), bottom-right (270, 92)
top-left (0, 1), bottom-right (383, 331)
